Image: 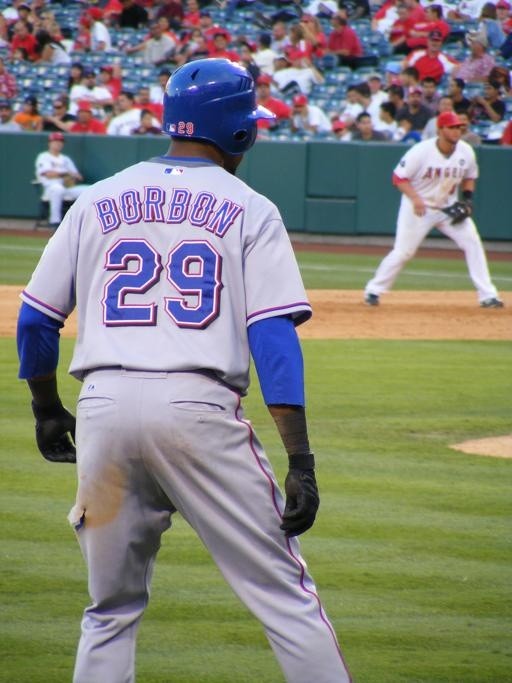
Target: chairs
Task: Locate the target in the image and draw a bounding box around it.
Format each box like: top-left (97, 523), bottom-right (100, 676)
top-left (2, 0), bottom-right (511, 144)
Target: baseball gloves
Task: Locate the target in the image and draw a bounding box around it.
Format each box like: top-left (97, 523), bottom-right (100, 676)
top-left (442, 199), bottom-right (472, 225)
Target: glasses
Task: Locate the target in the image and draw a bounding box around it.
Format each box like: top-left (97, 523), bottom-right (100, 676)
top-left (52, 104), bottom-right (65, 108)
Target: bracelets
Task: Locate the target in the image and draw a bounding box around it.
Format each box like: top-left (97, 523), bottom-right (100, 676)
top-left (462, 189), bottom-right (472, 201)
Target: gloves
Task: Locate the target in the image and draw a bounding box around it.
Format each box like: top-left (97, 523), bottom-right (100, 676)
top-left (279, 449), bottom-right (320, 538)
top-left (29, 393), bottom-right (82, 464)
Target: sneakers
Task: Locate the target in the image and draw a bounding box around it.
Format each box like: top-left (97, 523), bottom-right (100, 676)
top-left (479, 297), bottom-right (505, 309)
top-left (362, 288), bottom-right (380, 305)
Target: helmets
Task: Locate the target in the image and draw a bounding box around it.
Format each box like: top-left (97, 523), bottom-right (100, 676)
top-left (158, 56), bottom-right (259, 156)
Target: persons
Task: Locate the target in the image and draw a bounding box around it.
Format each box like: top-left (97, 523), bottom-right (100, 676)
top-left (1, 0), bottom-right (512, 147)
top-left (17, 55), bottom-right (353, 682)
top-left (34, 128), bottom-right (94, 228)
top-left (363, 111), bottom-right (507, 308)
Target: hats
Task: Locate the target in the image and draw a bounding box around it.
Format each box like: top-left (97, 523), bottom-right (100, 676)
top-left (436, 110), bottom-right (466, 127)
top-left (98, 64), bottom-right (112, 73)
top-left (15, 2), bottom-right (31, 11)
top-left (256, 73), bottom-right (346, 131)
top-left (408, 0), bottom-right (509, 96)
top-left (84, 6), bottom-right (102, 19)
top-left (46, 131), bottom-right (64, 140)
